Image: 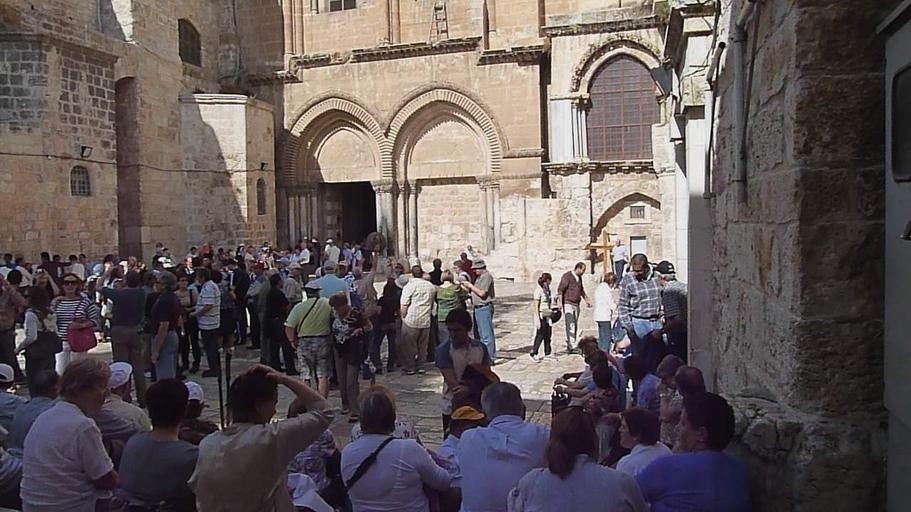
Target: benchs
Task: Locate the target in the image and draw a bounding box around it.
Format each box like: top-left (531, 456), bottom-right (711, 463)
top-left (563, 367), bottom-right (626, 413)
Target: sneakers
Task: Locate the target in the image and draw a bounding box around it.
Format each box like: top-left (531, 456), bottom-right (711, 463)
top-left (339, 407), bottom-right (350, 416)
top-left (565, 348), bottom-right (582, 354)
top-left (349, 412), bottom-right (360, 423)
top-left (528, 352), bottom-right (542, 363)
top-left (543, 354), bottom-right (560, 362)
top-left (405, 367), bottom-right (427, 375)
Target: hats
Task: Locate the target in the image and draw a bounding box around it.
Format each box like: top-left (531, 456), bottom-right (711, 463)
top-left (450, 404), bottom-right (485, 422)
top-left (471, 259), bottom-right (487, 271)
top-left (184, 379), bottom-right (205, 406)
top-left (324, 259), bottom-right (339, 272)
top-left (300, 280), bottom-right (324, 291)
top-left (0, 363), bottom-right (16, 384)
top-left (286, 261), bottom-right (305, 271)
top-left (653, 260), bottom-right (677, 274)
top-left (105, 361), bottom-right (133, 390)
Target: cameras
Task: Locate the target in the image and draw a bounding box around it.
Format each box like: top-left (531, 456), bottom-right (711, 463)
top-left (112, 268), bottom-right (119, 271)
top-left (36, 268), bottom-right (44, 273)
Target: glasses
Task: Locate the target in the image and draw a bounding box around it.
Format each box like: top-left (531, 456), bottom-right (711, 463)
top-left (63, 280), bottom-right (79, 287)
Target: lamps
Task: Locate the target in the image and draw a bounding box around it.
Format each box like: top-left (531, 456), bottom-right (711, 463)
top-left (82, 146), bottom-right (92, 158)
top-left (261, 163), bottom-right (268, 171)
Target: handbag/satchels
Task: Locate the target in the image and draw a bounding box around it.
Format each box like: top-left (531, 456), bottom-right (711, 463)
top-left (23, 310), bottom-right (63, 361)
top-left (67, 297), bottom-right (97, 353)
